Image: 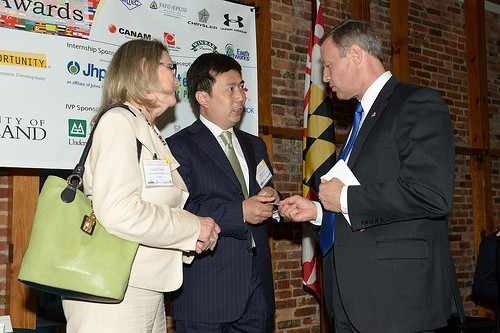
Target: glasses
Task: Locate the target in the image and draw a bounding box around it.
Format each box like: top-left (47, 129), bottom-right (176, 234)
top-left (158, 63), bottom-right (177, 77)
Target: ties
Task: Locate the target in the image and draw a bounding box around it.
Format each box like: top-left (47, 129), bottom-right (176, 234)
top-left (321, 104), bottom-right (364, 256)
top-left (219, 131), bottom-right (249, 200)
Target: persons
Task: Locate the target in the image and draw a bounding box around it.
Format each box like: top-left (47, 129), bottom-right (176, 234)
top-left (165, 53), bottom-right (283, 333)
top-left (471, 231), bottom-right (500, 333)
top-left (61, 38), bottom-right (220, 333)
top-left (276, 19), bottom-right (465, 333)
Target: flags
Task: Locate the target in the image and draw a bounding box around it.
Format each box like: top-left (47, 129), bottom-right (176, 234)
top-left (302, 0), bottom-right (336, 301)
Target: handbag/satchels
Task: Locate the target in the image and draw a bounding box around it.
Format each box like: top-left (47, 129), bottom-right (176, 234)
top-left (16, 103), bottom-right (144, 303)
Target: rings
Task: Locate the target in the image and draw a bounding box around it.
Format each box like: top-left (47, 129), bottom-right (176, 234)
top-left (210, 239), bottom-right (215, 242)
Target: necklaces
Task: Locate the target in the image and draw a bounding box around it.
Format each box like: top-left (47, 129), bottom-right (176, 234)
top-left (139, 109), bottom-right (167, 146)
